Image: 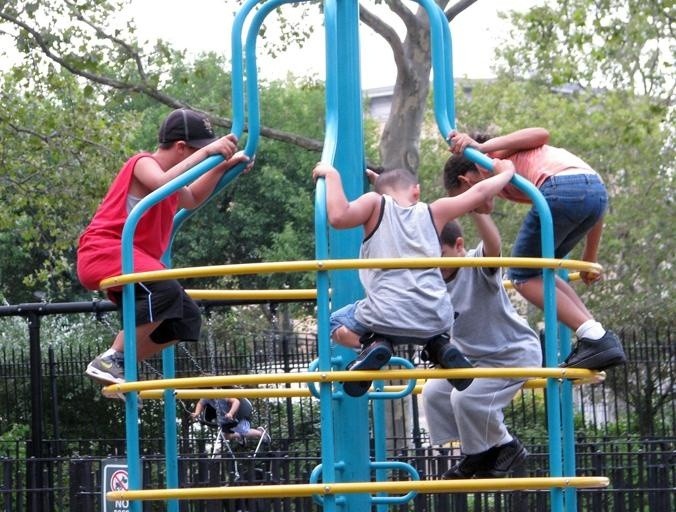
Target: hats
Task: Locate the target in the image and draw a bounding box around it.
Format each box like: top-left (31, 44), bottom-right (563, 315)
top-left (160, 107), bottom-right (219, 149)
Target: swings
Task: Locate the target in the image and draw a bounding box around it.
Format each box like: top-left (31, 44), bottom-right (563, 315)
top-left (88, 300), bottom-right (280, 485)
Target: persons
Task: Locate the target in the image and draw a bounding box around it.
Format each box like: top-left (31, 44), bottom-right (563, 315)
top-left (186, 371), bottom-right (272, 447)
top-left (419, 205), bottom-right (543, 481)
top-left (74, 104), bottom-right (258, 414)
top-left (311, 156), bottom-right (516, 400)
top-left (440, 122), bottom-right (630, 375)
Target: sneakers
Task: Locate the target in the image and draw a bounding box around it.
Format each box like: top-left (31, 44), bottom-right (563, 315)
top-left (558, 329), bottom-right (626, 369)
top-left (257, 426), bottom-right (271, 444)
top-left (344, 333), bottom-right (392, 396)
top-left (440, 435), bottom-right (529, 480)
top-left (422, 332), bottom-right (475, 391)
top-left (85, 348), bottom-right (144, 410)
